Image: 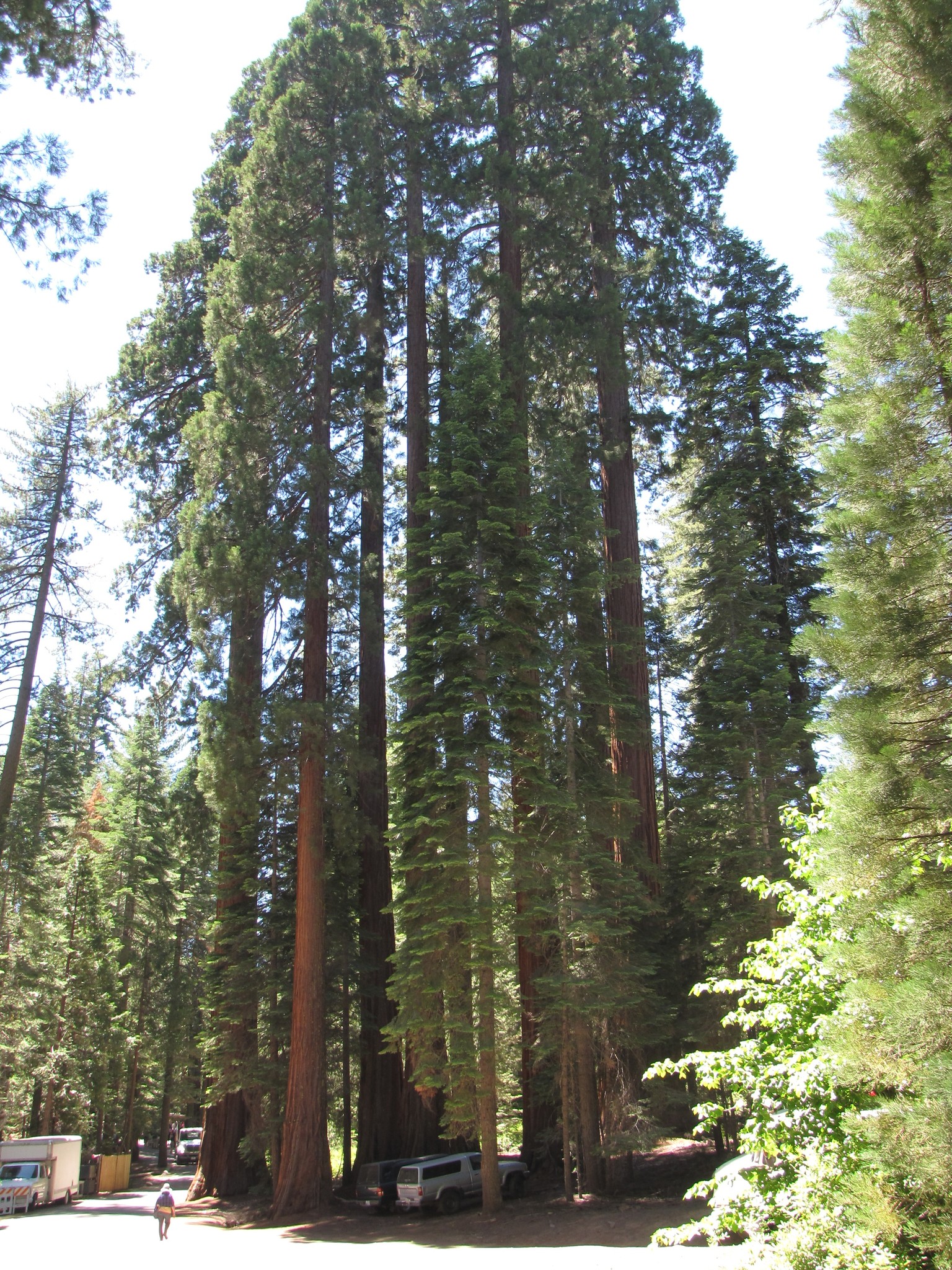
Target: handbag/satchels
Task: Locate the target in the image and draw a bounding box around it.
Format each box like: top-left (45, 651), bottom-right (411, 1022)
top-left (159, 1206), bottom-right (172, 1214)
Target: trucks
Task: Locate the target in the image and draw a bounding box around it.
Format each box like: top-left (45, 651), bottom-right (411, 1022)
top-left (0, 1135), bottom-right (82, 1211)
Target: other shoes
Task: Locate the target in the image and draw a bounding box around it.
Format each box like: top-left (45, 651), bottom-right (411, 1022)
top-left (160, 1235), bottom-right (163, 1240)
top-left (163, 1234), bottom-right (168, 1239)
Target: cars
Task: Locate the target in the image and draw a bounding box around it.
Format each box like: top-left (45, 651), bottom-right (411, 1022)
top-left (174, 1127), bottom-right (203, 1164)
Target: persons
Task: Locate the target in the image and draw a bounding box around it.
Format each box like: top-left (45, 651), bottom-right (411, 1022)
top-left (142, 1119), bottom-right (186, 1155)
top-left (154, 1183), bottom-right (176, 1243)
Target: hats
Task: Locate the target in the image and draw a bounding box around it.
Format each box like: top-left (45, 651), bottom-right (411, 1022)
top-left (160, 1183), bottom-right (172, 1192)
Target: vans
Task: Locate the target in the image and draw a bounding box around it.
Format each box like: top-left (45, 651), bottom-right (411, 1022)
top-left (355, 1152), bottom-right (530, 1217)
